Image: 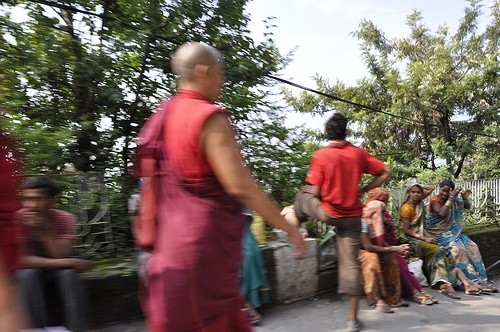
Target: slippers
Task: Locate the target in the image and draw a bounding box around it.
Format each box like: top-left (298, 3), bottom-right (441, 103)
top-left (374, 302), bottom-right (395, 313)
top-left (394, 297), bottom-right (409, 307)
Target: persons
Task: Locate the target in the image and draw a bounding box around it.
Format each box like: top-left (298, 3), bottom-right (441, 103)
top-left (428, 181), bottom-right (500, 292)
top-left (364, 187), bottom-right (438, 306)
top-left (358, 201), bottom-right (410, 313)
top-left (274, 113), bottom-right (394, 332)
top-left (0, 133), bottom-right (98, 332)
top-left (445, 246), bottom-right (492, 295)
top-left (400, 184), bottom-right (461, 299)
top-left (133, 41), bottom-right (308, 332)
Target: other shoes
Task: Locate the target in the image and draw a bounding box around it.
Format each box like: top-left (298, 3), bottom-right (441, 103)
top-left (465, 284), bottom-right (482, 296)
top-left (419, 290), bottom-right (438, 303)
top-left (440, 283), bottom-right (462, 300)
top-left (411, 292), bottom-right (433, 305)
top-left (244, 301), bottom-right (262, 323)
top-left (347, 318), bottom-right (364, 331)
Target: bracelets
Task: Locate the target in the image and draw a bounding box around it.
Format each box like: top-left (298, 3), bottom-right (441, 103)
top-left (445, 200), bottom-right (453, 207)
top-left (415, 233), bottom-right (420, 238)
top-left (450, 196), bottom-right (455, 200)
